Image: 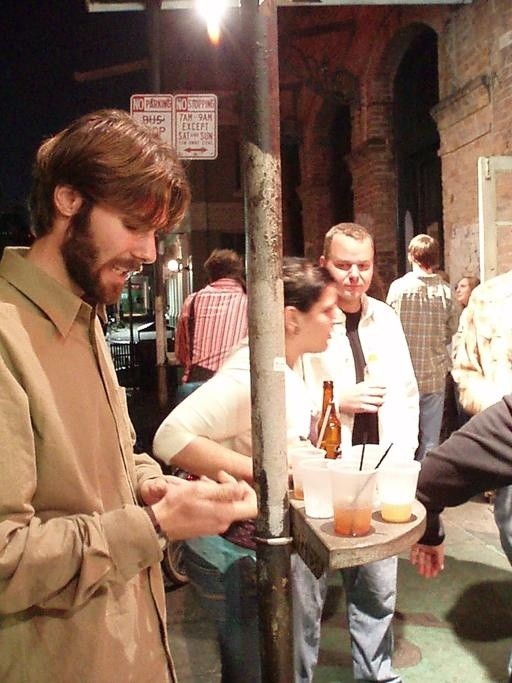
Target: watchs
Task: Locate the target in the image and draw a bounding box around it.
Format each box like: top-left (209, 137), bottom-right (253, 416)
top-left (144, 506), bottom-right (169, 550)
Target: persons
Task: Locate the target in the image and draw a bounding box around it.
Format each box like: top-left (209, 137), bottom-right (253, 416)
top-left (153, 258), bottom-right (336, 682)
top-left (450, 273), bottom-right (512, 564)
top-left (1, 110), bottom-right (259, 682)
top-left (456, 276), bottom-right (480, 308)
top-left (175, 248), bottom-right (249, 382)
top-left (291, 222), bottom-right (420, 682)
top-left (385, 234), bottom-right (459, 462)
top-left (411, 394), bottom-right (512, 576)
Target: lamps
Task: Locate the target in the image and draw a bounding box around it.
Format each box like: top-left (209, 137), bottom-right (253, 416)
top-left (167, 259), bottom-right (184, 272)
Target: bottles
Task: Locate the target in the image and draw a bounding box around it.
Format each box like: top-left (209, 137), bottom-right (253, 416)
top-left (316, 380), bottom-right (342, 460)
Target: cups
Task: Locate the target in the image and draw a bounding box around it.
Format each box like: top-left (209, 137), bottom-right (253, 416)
top-left (380, 460), bottom-right (423, 522)
top-left (290, 448), bottom-right (326, 499)
top-left (352, 445), bottom-right (383, 468)
top-left (326, 462), bottom-right (378, 535)
top-left (302, 458), bottom-right (338, 520)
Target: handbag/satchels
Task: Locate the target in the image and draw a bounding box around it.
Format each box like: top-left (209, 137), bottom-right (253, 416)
top-left (186, 293), bottom-right (216, 382)
